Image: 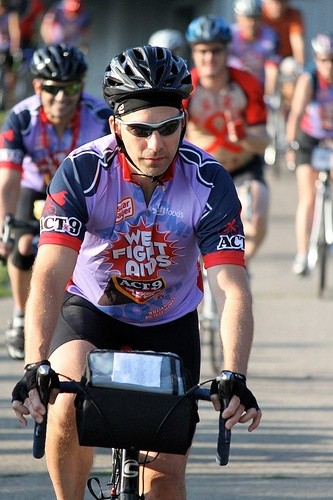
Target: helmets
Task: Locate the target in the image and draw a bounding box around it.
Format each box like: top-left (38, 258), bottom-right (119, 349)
top-left (30, 43), bottom-right (86, 81)
top-left (234, 0), bottom-right (261, 17)
top-left (148, 29), bottom-right (184, 51)
top-left (102, 45), bottom-right (193, 117)
top-left (311, 33), bottom-right (333, 58)
top-left (185, 15), bottom-right (232, 44)
top-left (63, 0), bottom-right (83, 19)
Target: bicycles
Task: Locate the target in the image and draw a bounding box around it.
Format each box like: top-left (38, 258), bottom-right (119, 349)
top-left (32, 349), bottom-right (232, 500)
top-left (285, 140), bottom-right (333, 298)
top-left (0, 65), bottom-right (303, 332)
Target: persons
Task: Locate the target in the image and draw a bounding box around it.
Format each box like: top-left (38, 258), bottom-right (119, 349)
top-left (0, 42), bottom-right (114, 360)
top-left (284, 33), bottom-right (333, 276)
top-left (11, 45), bottom-right (263, 500)
top-left (0, 0), bottom-right (306, 262)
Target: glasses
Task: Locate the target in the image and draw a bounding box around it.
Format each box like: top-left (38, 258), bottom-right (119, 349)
top-left (319, 57), bottom-right (333, 62)
top-left (39, 81), bottom-right (80, 96)
top-left (114, 111), bottom-right (184, 138)
top-left (201, 48), bottom-right (223, 54)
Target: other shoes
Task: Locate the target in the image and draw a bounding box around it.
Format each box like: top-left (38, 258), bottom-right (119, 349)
top-left (293, 256), bottom-right (308, 278)
top-left (6, 314), bottom-right (26, 360)
